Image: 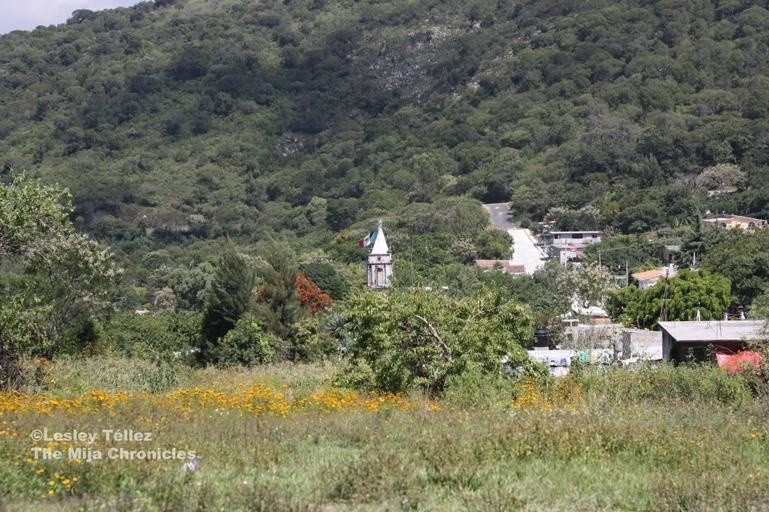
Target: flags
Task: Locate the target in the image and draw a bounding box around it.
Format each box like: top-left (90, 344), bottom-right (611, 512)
top-left (356, 230), bottom-right (379, 249)
